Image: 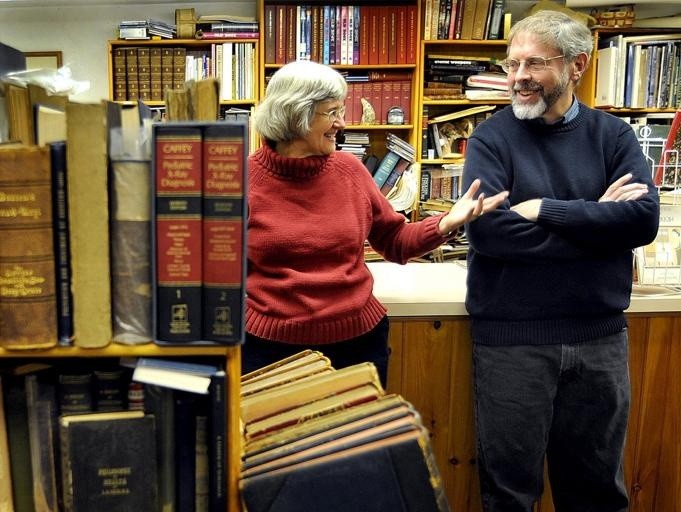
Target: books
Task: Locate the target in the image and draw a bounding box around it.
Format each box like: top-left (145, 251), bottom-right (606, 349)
top-left (617, 113), bottom-right (679, 185)
top-left (195, 15), bottom-right (258, 39)
top-left (119, 19), bottom-right (178, 40)
top-left (423, 54), bottom-right (511, 101)
top-left (0, 80), bottom-right (255, 351)
top-left (239, 349), bottom-right (452, 512)
top-left (364, 226), bottom-right (470, 268)
top-left (421, 105), bottom-right (497, 159)
top-left (418, 164), bottom-right (464, 222)
top-left (335, 132), bottom-right (416, 211)
top-left (631, 14), bottom-right (679, 27)
top-left (265, 67), bottom-right (412, 125)
top-left (420, 2), bottom-right (512, 40)
top-left (113, 43), bottom-right (255, 101)
top-left (573, 32), bottom-right (679, 109)
top-left (264, 4), bottom-right (418, 65)
top-left (1, 357), bottom-right (229, 508)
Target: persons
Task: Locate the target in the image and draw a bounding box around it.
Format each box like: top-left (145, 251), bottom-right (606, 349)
top-left (238, 63), bottom-right (471, 391)
top-left (238, 58), bottom-right (512, 391)
top-left (459, 8), bottom-right (661, 510)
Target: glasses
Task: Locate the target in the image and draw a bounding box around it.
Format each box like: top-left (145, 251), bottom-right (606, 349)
top-left (501, 48), bottom-right (573, 72)
top-left (308, 106), bottom-right (347, 123)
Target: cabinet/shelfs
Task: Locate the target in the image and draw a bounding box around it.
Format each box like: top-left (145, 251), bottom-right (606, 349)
top-left (1, 346), bottom-right (244, 512)
top-left (109, 0), bottom-right (258, 124)
top-left (577, 26), bottom-right (679, 115)
top-left (424, 0), bottom-right (514, 259)
top-left (261, 2), bottom-right (421, 260)
